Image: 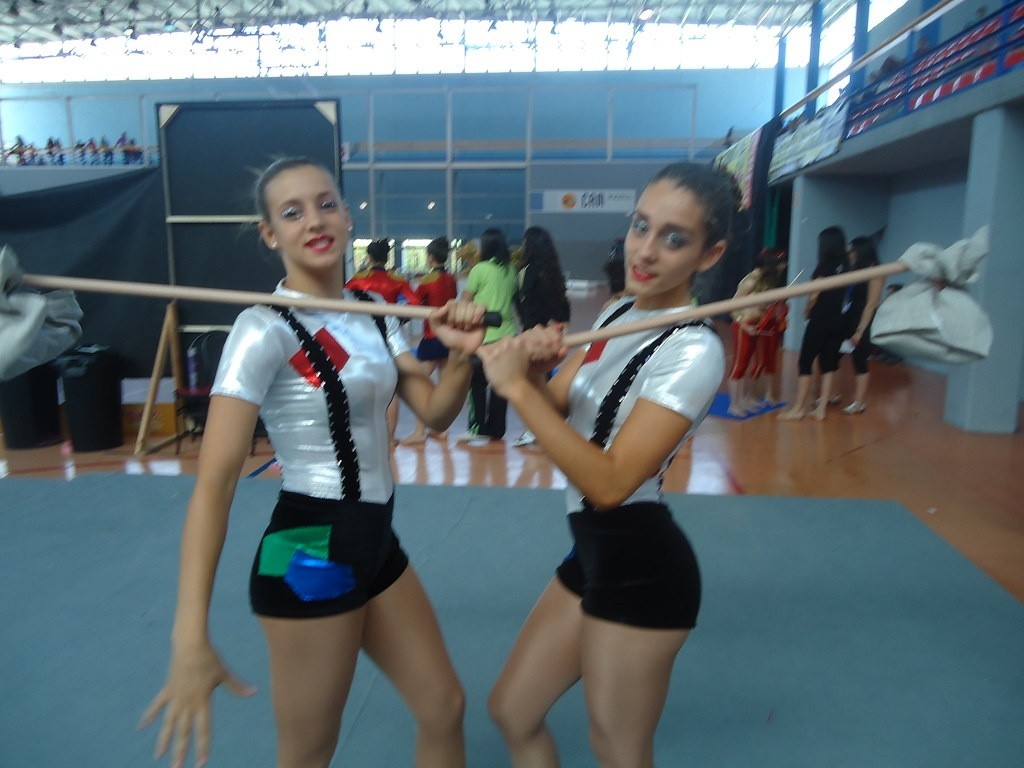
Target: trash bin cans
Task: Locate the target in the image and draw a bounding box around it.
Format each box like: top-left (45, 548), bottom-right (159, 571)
top-left (51, 345), bottom-right (125, 452)
top-left (0, 358), bottom-right (64, 449)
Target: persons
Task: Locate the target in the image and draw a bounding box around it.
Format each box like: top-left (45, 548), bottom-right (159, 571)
top-left (132, 152), bottom-right (488, 767)
top-left (599, 224), bottom-right (883, 422)
top-left (474, 162), bottom-right (744, 767)
top-left (342, 224), bottom-right (574, 446)
top-left (5, 132), bottom-right (142, 170)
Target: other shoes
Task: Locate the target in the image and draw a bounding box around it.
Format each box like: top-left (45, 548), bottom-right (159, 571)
top-left (815, 392), bottom-right (836, 406)
top-left (466, 435), bottom-right (492, 448)
top-left (429, 430), bottom-right (447, 439)
top-left (513, 429), bottom-right (536, 446)
top-left (457, 433), bottom-right (492, 440)
top-left (776, 410), bottom-right (805, 420)
top-left (763, 399), bottom-right (777, 407)
top-left (808, 410), bottom-right (826, 420)
top-left (727, 407), bottom-right (746, 417)
top-left (745, 398), bottom-right (761, 408)
top-left (842, 401), bottom-right (865, 413)
top-left (403, 434), bottom-right (425, 446)
